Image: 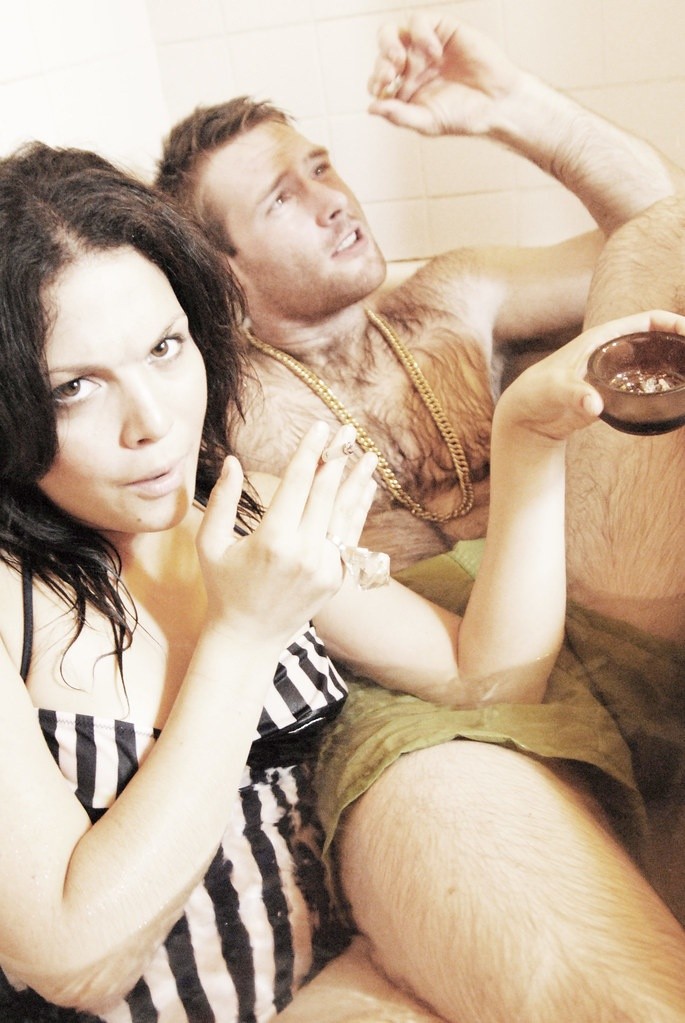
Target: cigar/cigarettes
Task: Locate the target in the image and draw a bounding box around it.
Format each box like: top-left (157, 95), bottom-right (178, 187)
top-left (319, 443), bottom-right (354, 465)
top-left (377, 78), bottom-right (400, 101)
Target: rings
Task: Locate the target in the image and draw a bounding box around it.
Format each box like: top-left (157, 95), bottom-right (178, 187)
top-left (325, 532), bottom-right (389, 589)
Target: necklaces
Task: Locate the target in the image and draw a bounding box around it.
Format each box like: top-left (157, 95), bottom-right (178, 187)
top-left (246, 305), bottom-right (475, 522)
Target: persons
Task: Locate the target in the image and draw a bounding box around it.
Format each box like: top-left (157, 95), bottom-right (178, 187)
top-left (156, 18), bottom-right (685, 1023)
top-left (0, 140), bottom-right (683, 1023)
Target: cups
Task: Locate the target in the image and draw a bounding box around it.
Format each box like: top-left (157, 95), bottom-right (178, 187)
top-left (586, 331), bottom-right (685, 431)
